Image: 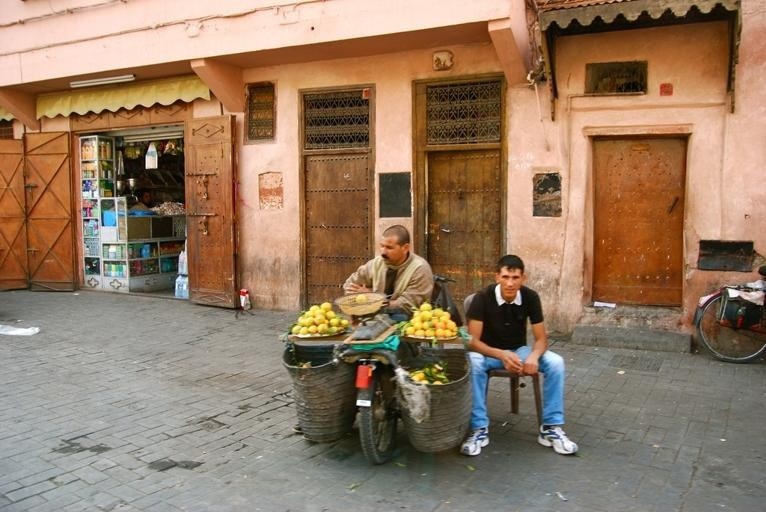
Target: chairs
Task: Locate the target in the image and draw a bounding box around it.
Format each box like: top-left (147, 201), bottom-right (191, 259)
top-left (464, 294), bottom-right (543, 430)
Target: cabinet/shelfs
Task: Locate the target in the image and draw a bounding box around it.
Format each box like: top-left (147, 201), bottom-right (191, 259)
top-left (128, 214), bottom-right (185, 293)
top-left (126, 161), bottom-right (184, 207)
top-left (80, 136), bottom-right (128, 294)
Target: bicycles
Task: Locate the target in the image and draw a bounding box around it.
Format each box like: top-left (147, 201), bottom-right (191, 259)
top-left (693, 265), bottom-right (765, 363)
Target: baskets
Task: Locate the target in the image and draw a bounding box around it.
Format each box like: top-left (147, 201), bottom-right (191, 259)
top-left (334, 292), bottom-right (383, 316)
top-left (395, 347), bottom-right (472, 453)
top-left (281, 346), bottom-right (357, 442)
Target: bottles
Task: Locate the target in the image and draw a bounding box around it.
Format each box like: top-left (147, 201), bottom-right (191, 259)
top-left (81, 141), bottom-right (112, 160)
top-left (82, 161), bottom-right (114, 178)
top-left (174, 275), bottom-right (189, 297)
top-left (177, 250), bottom-right (188, 273)
top-left (82, 180), bottom-right (106, 199)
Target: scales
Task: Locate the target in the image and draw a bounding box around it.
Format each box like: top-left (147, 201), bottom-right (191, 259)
top-left (334, 291), bottom-right (390, 341)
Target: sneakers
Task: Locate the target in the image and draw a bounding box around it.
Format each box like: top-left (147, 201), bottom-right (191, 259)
top-left (460, 427), bottom-right (489, 456)
top-left (537, 425), bottom-right (578, 455)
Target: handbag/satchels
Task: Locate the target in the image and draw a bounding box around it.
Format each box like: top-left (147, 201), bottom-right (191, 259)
top-left (720, 288), bottom-right (766, 332)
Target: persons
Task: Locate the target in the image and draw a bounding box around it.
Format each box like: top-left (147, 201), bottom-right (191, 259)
top-left (460, 255), bottom-right (578, 457)
top-left (130, 189), bottom-right (157, 210)
top-left (344, 224), bottom-right (433, 321)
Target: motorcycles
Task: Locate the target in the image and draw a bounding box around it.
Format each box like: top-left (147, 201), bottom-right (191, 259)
top-left (279, 275), bottom-right (470, 465)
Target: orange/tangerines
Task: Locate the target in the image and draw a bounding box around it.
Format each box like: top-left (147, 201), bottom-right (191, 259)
top-left (356, 294), bottom-right (368, 304)
top-left (403, 301), bottom-right (458, 338)
top-left (410, 363), bottom-right (450, 385)
top-left (291, 301), bottom-right (348, 335)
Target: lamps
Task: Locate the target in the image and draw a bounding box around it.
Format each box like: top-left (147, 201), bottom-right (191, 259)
top-left (70, 74), bottom-right (136, 89)
